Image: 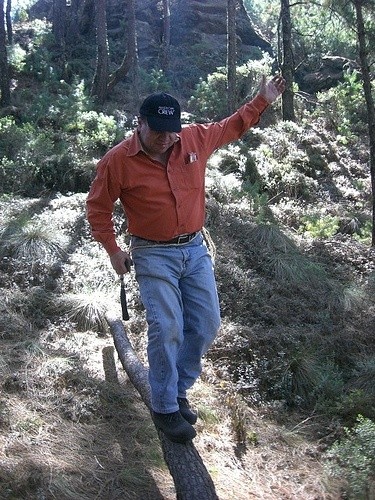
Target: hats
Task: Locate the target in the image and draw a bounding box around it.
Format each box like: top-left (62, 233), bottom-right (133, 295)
top-left (139, 93), bottom-right (183, 134)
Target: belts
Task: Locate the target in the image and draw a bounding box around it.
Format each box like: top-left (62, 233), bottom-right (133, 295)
top-left (134, 233), bottom-right (200, 246)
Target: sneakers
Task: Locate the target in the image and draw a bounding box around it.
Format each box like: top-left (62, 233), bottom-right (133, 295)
top-left (176, 393), bottom-right (198, 425)
top-left (153, 408), bottom-right (198, 443)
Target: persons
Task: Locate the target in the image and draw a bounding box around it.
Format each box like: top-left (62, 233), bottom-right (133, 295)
top-left (86, 73), bottom-right (287, 442)
